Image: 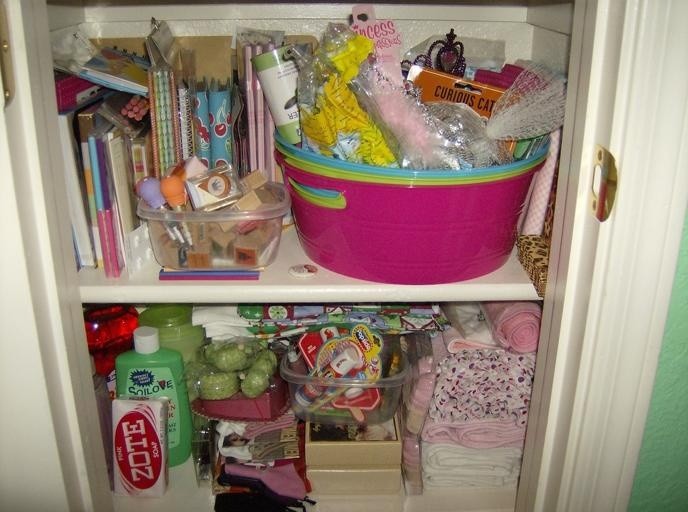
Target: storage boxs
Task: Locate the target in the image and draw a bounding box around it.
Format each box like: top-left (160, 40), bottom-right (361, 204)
top-left (303, 410), bottom-right (407, 511)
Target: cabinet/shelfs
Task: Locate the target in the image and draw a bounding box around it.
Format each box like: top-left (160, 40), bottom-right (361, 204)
top-left (16, 0), bottom-right (688, 512)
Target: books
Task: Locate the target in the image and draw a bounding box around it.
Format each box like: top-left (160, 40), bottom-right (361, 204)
top-left (55, 30), bottom-right (280, 281)
